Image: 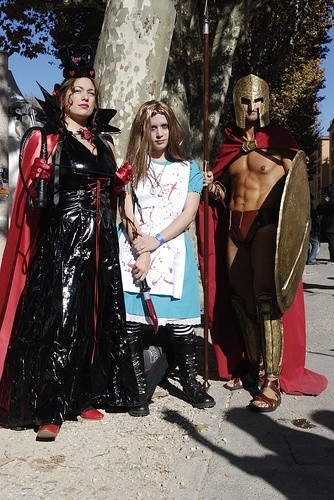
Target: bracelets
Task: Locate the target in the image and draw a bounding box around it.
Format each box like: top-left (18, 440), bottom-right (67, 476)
top-left (155, 234), bottom-right (164, 244)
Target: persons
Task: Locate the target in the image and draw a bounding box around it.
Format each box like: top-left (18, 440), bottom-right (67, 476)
top-left (196, 73), bottom-right (327, 413)
top-left (0, 76), bottom-right (141, 438)
top-left (115, 100), bottom-right (216, 418)
top-left (306, 190), bottom-right (334, 264)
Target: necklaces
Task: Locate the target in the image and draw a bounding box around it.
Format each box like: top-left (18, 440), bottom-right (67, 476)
top-left (152, 159), bottom-right (169, 186)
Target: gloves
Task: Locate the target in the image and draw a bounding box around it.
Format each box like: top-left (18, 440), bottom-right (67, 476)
top-left (115, 161), bottom-right (134, 195)
top-left (30, 157), bottom-right (55, 191)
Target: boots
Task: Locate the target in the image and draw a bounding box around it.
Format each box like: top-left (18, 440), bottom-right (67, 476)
top-left (124, 329), bottom-right (150, 418)
top-left (172, 332), bottom-right (216, 409)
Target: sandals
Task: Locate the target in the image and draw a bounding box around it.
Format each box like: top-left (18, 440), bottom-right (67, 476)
top-left (224, 364), bottom-right (266, 391)
top-left (248, 381), bottom-right (282, 413)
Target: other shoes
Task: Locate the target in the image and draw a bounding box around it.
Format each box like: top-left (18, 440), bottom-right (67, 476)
top-left (37, 424), bottom-right (60, 439)
top-left (80, 407), bottom-right (104, 421)
top-left (308, 260), bottom-right (319, 265)
